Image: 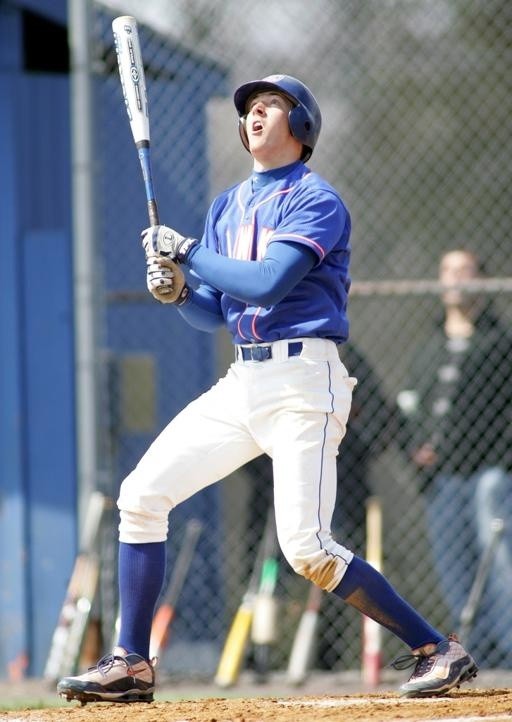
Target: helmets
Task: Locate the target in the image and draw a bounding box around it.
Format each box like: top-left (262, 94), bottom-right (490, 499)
top-left (234, 74), bottom-right (321, 164)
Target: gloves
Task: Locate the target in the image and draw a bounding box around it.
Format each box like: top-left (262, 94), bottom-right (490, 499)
top-left (141, 225), bottom-right (198, 264)
top-left (146, 257), bottom-right (189, 308)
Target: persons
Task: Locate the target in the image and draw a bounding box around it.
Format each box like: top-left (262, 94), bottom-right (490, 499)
top-left (239, 341), bottom-right (417, 683)
top-left (399, 247), bottom-right (512, 669)
top-left (56, 72), bottom-right (478, 710)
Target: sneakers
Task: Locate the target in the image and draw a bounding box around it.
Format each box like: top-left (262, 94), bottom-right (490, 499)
top-left (390, 633), bottom-right (479, 698)
top-left (57, 645), bottom-right (155, 704)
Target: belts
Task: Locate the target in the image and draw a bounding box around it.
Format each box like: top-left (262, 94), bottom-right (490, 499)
top-left (236, 342), bottom-right (303, 361)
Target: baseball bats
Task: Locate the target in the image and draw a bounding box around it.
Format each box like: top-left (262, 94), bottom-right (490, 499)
top-left (286, 584), bottom-right (324, 687)
top-left (111, 15), bottom-right (171, 294)
top-left (214, 504), bottom-right (279, 694)
top-left (459, 516), bottom-right (504, 646)
top-left (148, 517), bottom-right (204, 666)
top-left (363, 495), bottom-right (383, 691)
top-left (42, 489), bottom-right (106, 692)
